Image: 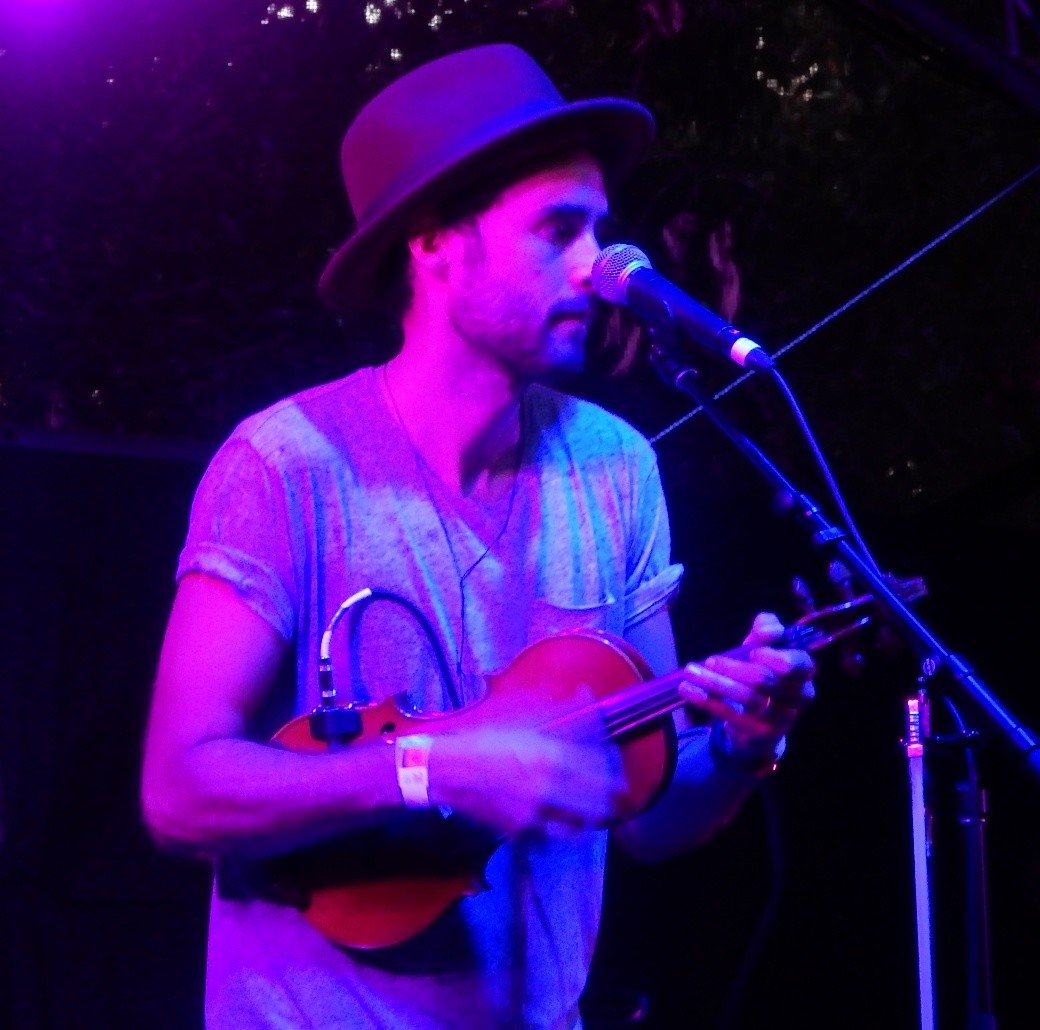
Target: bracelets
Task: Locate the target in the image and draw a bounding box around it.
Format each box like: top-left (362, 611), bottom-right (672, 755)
top-left (711, 718), bottom-right (787, 778)
top-left (396, 732), bottom-right (451, 821)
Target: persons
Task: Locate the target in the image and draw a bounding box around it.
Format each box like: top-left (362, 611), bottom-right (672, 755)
top-left (598, 174), bottom-right (765, 378)
top-left (140, 44), bottom-right (818, 1030)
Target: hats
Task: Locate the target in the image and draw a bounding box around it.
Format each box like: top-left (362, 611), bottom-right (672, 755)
top-left (312, 40), bottom-right (657, 309)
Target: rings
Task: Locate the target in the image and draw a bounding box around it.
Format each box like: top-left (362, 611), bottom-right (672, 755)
top-left (754, 695), bottom-right (772, 719)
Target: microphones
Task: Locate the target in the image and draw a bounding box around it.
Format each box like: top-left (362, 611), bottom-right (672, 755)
top-left (592, 243), bottom-right (777, 377)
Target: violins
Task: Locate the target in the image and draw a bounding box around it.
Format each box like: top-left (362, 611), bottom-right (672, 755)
top-left (263, 559), bottom-right (929, 952)
